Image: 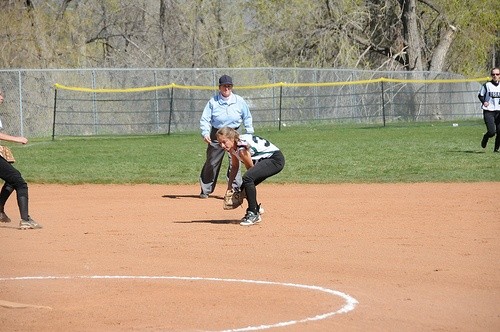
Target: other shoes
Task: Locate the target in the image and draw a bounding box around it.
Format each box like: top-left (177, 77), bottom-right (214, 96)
top-left (481, 136), bottom-right (489, 148)
top-left (19, 215), bottom-right (42, 229)
top-left (0, 212), bottom-right (11, 223)
top-left (259, 203), bottom-right (264, 214)
top-left (200, 193), bottom-right (209, 199)
top-left (494, 149), bottom-right (500, 152)
top-left (240, 208), bottom-right (261, 226)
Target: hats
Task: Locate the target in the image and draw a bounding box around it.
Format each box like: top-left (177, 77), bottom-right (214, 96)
top-left (219, 75), bottom-right (233, 86)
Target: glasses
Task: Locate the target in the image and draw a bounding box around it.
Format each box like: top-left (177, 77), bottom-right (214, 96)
top-left (491, 73), bottom-right (500, 76)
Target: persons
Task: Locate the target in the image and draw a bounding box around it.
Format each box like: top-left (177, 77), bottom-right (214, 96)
top-left (200, 74), bottom-right (253, 198)
top-left (478, 68), bottom-right (500, 152)
top-left (216, 127), bottom-right (285, 226)
top-left (0, 87), bottom-right (39, 230)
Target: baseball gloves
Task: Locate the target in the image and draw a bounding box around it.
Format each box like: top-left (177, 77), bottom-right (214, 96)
top-left (0, 146), bottom-right (15, 163)
top-left (224, 189), bottom-right (244, 210)
